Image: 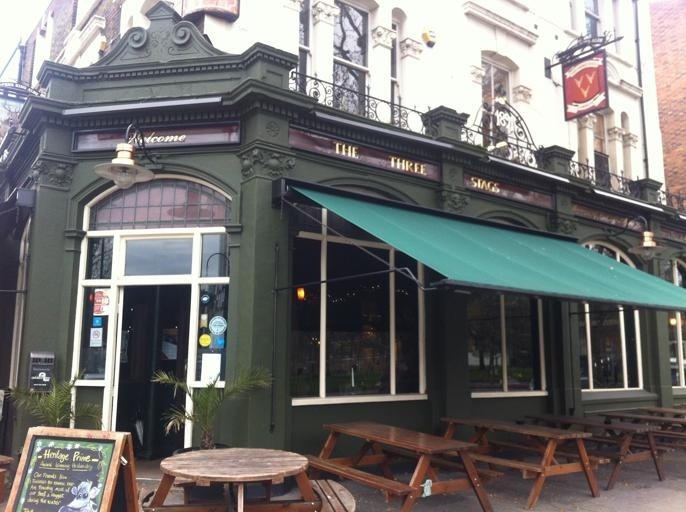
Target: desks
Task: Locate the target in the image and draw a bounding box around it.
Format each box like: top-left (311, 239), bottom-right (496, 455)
top-left (439, 407), bottom-right (686, 511)
top-left (150, 446), bottom-right (315, 511)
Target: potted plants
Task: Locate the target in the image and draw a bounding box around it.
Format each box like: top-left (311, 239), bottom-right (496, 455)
top-left (148, 365), bottom-right (273, 500)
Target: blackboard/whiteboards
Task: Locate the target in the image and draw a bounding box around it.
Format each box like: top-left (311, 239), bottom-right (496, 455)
top-left (4, 427), bottom-right (126, 512)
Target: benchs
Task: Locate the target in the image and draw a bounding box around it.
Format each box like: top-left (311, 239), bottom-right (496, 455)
top-left (308, 421), bottom-right (686, 512)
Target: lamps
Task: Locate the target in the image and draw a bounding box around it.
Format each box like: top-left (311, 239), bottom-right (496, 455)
top-left (604, 211), bottom-right (667, 260)
top-left (92, 123), bottom-right (155, 190)
